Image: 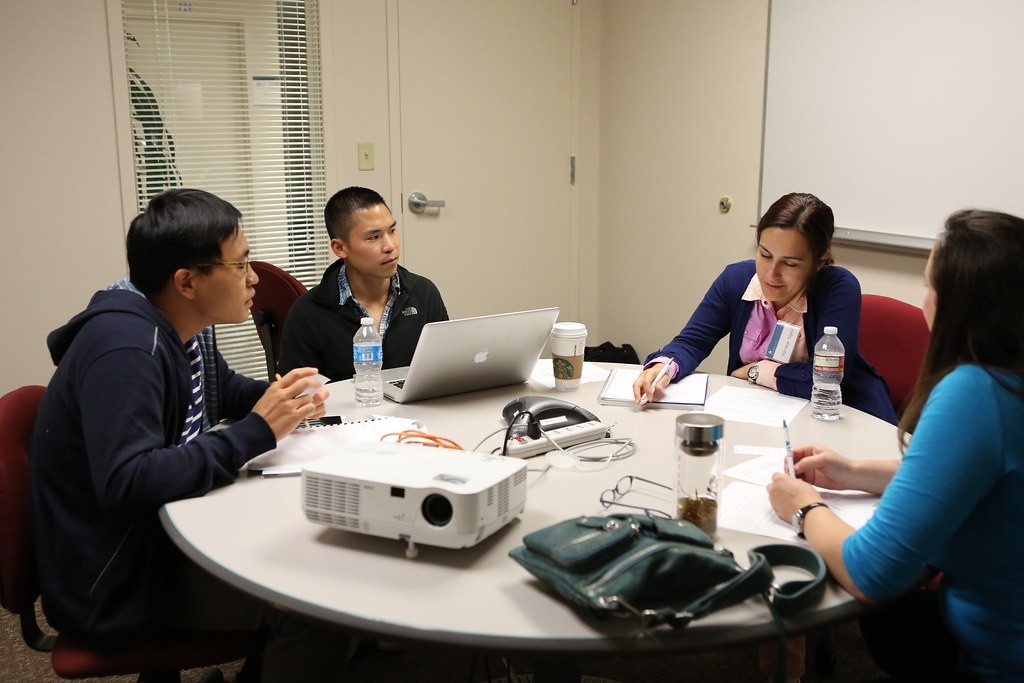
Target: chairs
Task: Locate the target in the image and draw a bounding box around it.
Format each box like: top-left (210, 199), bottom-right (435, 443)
top-left (857, 294), bottom-right (931, 413)
top-left (1, 384), bottom-right (267, 683)
top-left (247, 261), bottom-right (307, 382)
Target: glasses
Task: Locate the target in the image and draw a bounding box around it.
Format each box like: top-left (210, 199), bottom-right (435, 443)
top-left (600, 475), bottom-right (676, 518)
top-left (187, 254), bottom-right (249, 273)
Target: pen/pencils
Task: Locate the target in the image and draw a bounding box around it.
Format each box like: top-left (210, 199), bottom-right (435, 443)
top-left (638, 357), bottom-right (675, 406)
top-left (274, 374), bottom-right (310, 429)
top-left (782, 420), bottom-right (796, 478)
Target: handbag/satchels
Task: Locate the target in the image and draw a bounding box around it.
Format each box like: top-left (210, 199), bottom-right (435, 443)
top-left (508, 512), bottom-right (828, 630)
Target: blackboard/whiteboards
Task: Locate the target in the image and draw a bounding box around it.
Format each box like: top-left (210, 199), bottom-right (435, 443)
top-left (750, 0), bottom-right (1024, 257)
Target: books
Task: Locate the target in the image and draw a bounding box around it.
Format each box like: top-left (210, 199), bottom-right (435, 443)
top-left (248, 417), bottom-right (425, 476)
top-left (597, 368), bottom-right (709, 411)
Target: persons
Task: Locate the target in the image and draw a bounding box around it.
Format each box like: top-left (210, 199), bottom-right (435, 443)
top-left (278, 185), bottom-right (449, 385)
top-left (31, 188), bottom-right (355, 683)
top-left (633, 192), bottom-right (899, 425)
top-left (767, 210), bottom-right (1024, 683)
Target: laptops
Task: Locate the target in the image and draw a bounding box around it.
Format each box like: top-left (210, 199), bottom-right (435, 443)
top-left (352, 307), bottom-right (561, 403)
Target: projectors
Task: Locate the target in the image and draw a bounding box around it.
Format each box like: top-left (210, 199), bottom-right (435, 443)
top-left (301, 440), bottom-right (528, 549)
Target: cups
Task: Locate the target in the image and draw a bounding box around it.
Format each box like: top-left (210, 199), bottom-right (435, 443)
top-left (550, 322), bottom-right (587, 388)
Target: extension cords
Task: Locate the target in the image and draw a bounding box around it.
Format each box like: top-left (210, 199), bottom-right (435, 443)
top-left (501, 419), bottom-right (606, 459)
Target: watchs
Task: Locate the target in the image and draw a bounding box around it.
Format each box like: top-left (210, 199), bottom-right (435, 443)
top-left (792, 502), bottom-right (829, 540)
top-left (747, 362), bottom-right (759, 384)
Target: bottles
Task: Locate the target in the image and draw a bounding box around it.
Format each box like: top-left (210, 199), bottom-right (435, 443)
top-left (810, 326), bottom-right (844, 422)
top-left (672, 413), bottom-right (724, 544)
top-left (351, 317), bottom-right (384, 407)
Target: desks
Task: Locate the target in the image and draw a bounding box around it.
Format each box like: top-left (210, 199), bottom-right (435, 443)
top-left (159, 358), bottom-right (916, 683)
